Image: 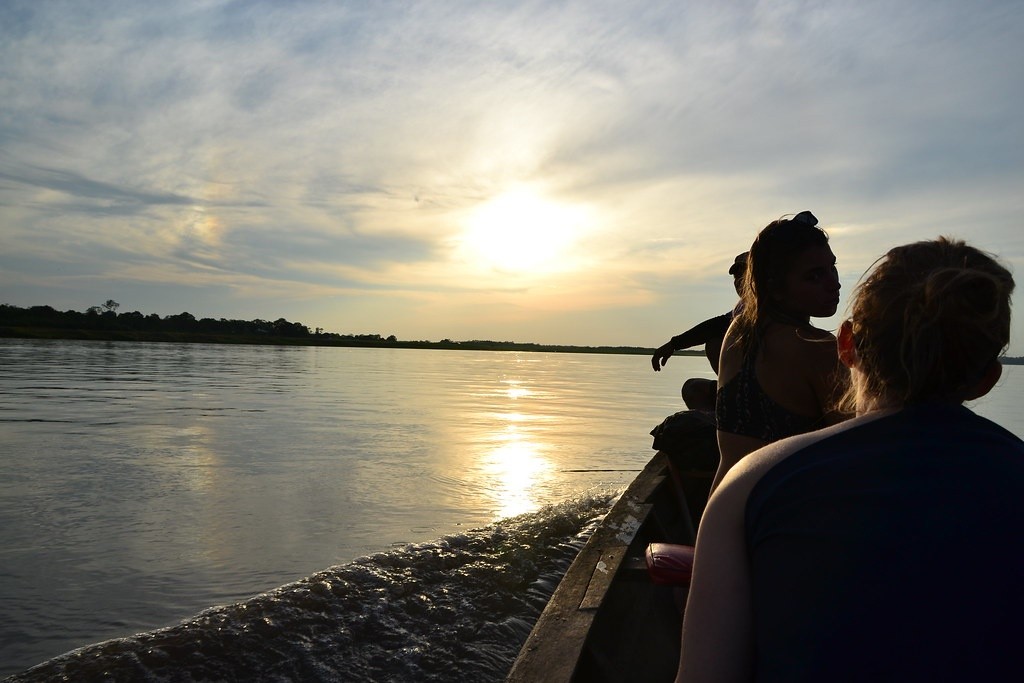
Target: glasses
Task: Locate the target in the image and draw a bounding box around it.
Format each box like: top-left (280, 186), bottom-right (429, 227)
top-left (774, 209), bottom-right (818, 253)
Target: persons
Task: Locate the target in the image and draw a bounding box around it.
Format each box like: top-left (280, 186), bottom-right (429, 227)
top-left (679, 238), bottom-right (1024, 683)
top-left (650, 252), bottom-right (748, 410)
top-left (708, 210), bottom-right (856, 505)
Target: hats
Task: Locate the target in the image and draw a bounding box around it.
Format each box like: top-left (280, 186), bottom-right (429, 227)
top-left (729, 251), bottom-right (750, 276)
